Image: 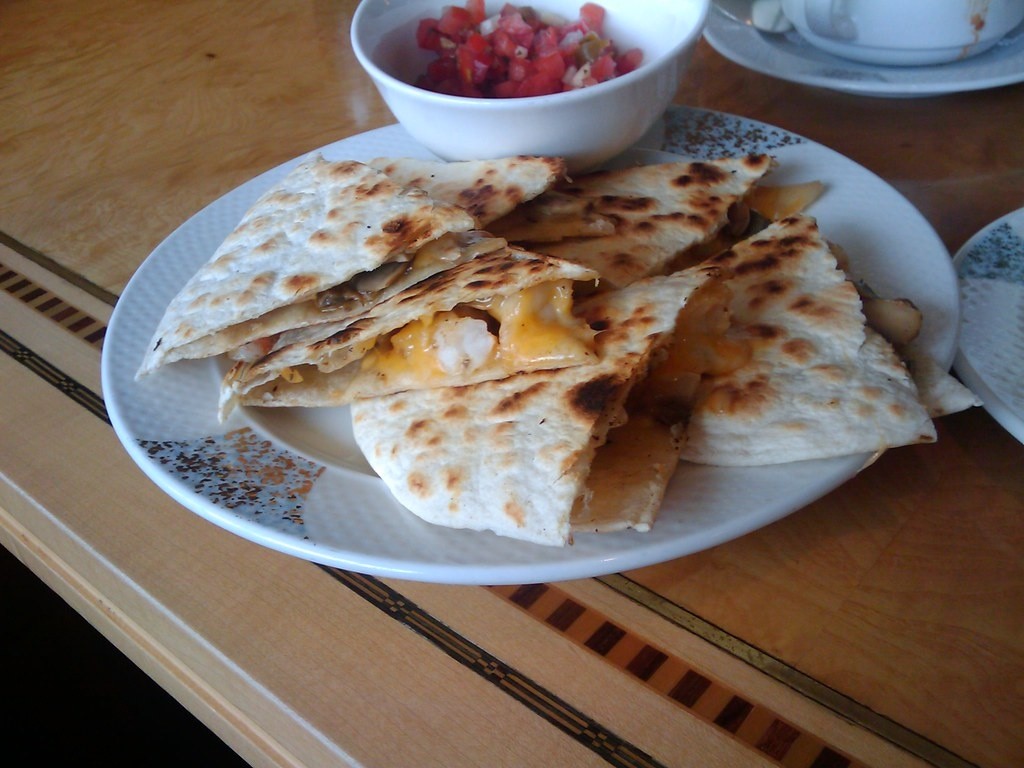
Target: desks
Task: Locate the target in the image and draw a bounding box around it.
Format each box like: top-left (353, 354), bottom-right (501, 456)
top-left (0, 0), bottom-right (1024, 768)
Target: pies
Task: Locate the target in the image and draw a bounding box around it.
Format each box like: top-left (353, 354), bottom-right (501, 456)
top-left (131, 151), bottom-right (990, 548)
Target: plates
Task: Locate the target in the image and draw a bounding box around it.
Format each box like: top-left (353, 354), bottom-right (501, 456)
top-left (699, 0), bottom-right (1024, 98)
top-left (102, 106), bottom-right (961, 583)
top-left (950, 204), bottom-right (1024, 447)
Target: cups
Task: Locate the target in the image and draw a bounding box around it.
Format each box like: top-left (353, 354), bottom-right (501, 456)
top-left (777, 0), bottom-right (1024, 67)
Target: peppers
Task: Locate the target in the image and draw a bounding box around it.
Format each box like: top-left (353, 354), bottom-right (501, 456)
top-left (417, 0), bottom-right (644, 100)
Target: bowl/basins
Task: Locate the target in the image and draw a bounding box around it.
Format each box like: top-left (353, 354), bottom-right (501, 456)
top-left (350, 0), bottom-right (709, 171)
